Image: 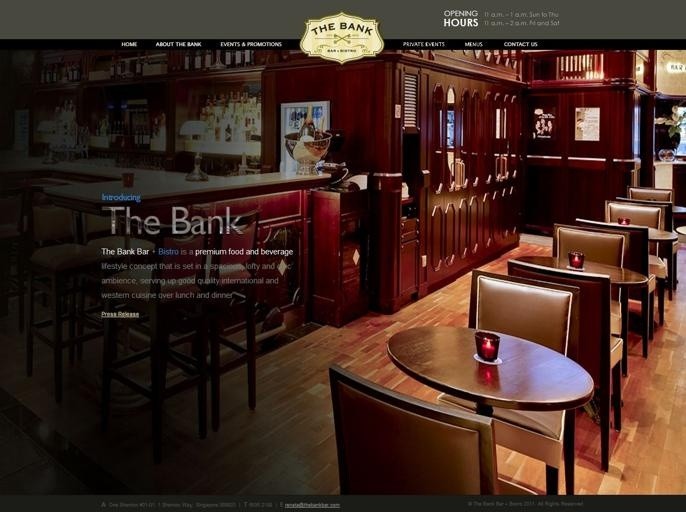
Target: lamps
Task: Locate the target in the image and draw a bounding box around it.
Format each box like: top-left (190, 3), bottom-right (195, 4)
top-left (179, 120), bottom-right (209, 182)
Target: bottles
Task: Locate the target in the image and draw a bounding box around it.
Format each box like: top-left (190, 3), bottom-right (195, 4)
top-left (51, 98), bottom-right (151, 148)
top-left (296, 105), bottom-right (316, 142)
top-left (39, 59), bottom-right (80, 83)
top-left (196, 87), bottom-right (261, 144)
top-left (288, 107), bottom-right (324, 130)
top-left (108, 47), bottom-right (257, 78)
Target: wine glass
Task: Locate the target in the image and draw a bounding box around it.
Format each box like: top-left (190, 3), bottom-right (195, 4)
top-left (284, 131), bottom-right (333, 177)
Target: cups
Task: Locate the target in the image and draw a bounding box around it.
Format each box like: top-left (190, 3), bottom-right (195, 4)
top-left (567, 250), bottom-right (585, 268)
top-left (473, 331), bottom-right (500, 363)
top-left (120, 172), bottom-right (135, 188)
top-left (658, 148), bottom-right (675, 162)
top-left (616, 216), bottom-right (632, 226)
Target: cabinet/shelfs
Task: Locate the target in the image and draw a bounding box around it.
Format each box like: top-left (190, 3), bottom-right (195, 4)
top-left (28, 49), bottom-right (278, 177)
top-left (372, 51), bottom-right (528, 314)
top-left (310, 189), bottom-right (371, 328)
